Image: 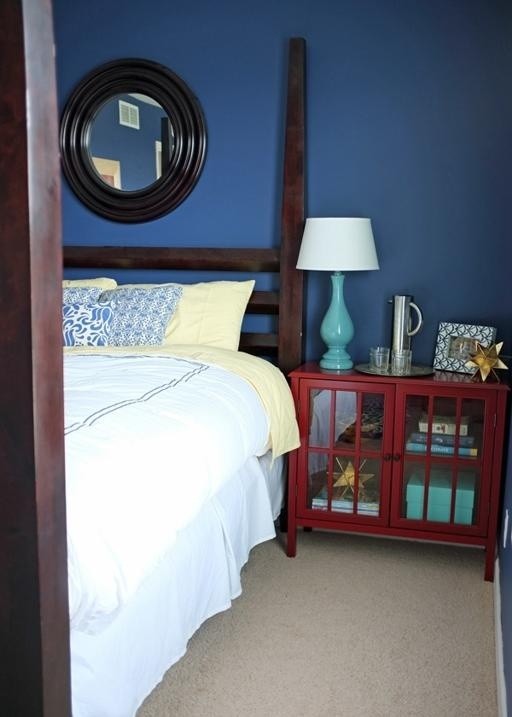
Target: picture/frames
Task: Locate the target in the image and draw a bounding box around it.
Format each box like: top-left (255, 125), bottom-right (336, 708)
top-left (434, 321), bottom-right (496, 378)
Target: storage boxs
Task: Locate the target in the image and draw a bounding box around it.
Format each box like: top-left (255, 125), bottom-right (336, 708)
top-left (404, 470), bottom-right (477, 526)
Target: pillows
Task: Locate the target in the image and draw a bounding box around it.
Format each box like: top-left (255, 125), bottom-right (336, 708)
top-left (61, 278), bottom-right (257, 350)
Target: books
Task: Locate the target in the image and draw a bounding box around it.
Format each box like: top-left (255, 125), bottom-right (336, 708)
top-left (418, 411), bottom-right (472, 436)
top-left (311, 504), bottom-right (380, 517)
top-left (409, 423), bottom-right (478, 447)
top-left (310, 479), bottom-right (380, 511)
top-left (405, 429), bottom-right (482, 458)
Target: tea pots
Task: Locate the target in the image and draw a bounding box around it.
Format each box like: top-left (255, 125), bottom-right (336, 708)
top-left (386, 294), bottom-right (423, 366)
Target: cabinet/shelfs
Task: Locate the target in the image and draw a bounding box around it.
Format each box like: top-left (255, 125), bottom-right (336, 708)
top-left (286, 360), bottom-right (508, 581)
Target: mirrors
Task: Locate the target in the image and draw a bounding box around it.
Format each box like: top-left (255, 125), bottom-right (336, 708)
top-left (56, 58), bottom-right (207, 224)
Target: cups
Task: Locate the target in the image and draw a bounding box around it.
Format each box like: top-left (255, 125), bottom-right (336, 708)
top-left (369, 347), bottom-right (389, 371)
top-left (392, 350), bottom-right (413, 372)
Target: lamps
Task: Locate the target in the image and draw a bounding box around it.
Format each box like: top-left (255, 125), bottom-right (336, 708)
top-left (295, 216), bottom-right (379, 370)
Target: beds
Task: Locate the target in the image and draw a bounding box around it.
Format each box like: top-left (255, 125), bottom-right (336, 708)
top-left (1, 248), bottom-right (305, 715)
